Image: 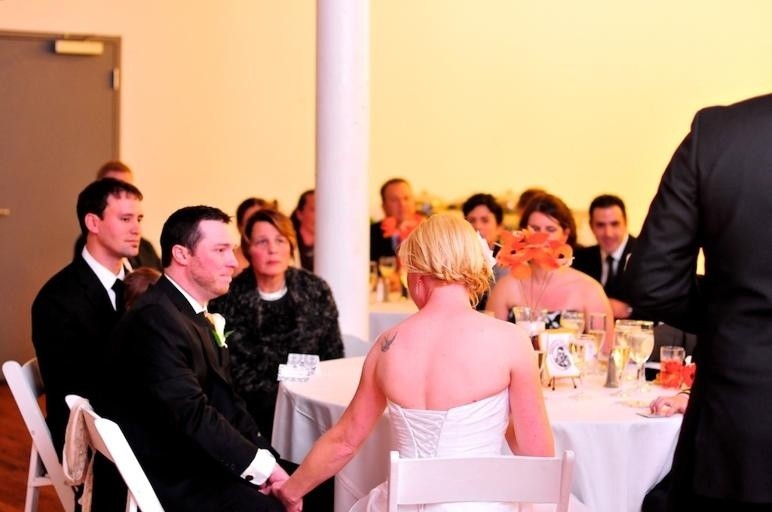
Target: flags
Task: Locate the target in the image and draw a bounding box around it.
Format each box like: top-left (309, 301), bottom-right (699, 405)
top-left (513, 307), bottom-right (545, 383)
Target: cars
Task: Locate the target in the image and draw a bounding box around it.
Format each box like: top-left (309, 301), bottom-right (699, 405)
top-left (661, 347), bottom-right (685, 394)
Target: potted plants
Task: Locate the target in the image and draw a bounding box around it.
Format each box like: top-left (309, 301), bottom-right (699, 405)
top-left (539, 328), bottom-right (582, 380)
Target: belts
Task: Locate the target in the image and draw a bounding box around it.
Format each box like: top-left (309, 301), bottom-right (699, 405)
top-left (611, 329), bottom-right (632, 396)
top-left (614, 320), bottom-right (655, 395)
top-left (569, 334), bottom-right (594, 390)
top-left (479, 307), bottom-right (497, 316)
top-left (589, 314), bottom-right (607, 371)
top-left (560, 311), bottom-right (584, 333)
top-left (512, 307), bottom-right (531, 326)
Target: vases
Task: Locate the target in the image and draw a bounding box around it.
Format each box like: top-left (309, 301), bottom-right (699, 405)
top-left (382, 202), bottom-right (434, 241)
top-left (487, 228), bottom-right (574, 307)
top-left (650, 357), bottom-right (696, 389)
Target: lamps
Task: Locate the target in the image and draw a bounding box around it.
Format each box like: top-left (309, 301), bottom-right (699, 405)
top-left (388, 450), bottom-right (575, 512)
top-left (66, 395), bottom-right (165, 512)
top-left (1, 358), bottom-right (76, 512)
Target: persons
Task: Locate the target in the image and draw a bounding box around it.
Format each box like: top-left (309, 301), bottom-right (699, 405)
top-left (573, 193), bottom-right (638, 319)
top-left (262, 212), bottom-right (560, 512)
top-left (232, 197), bottom-right (297, 280)
top-left (29, 178), bottom-right (141, 512)
top-left (142, 204), bottom-right (301, 511)
top-left (217, 210), bottom-right (345, 426)
top-left (626, 92), bottom-right (770, 512)
top-left (74, 160), bottom-right (162, 268)
top-left (463, 192), bottom-right (506, 258)
top-left (291, 189), bottom-right (316, 273)
top-left (370, 179), bottom-right (416, 259)
top-left (486, 192), bottom-right (615, 354)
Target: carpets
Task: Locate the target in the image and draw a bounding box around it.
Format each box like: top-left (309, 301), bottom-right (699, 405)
top-left (604, 255), bottom-right (615, 293)
top-left (111, 279), bottom-right (125, 315)
